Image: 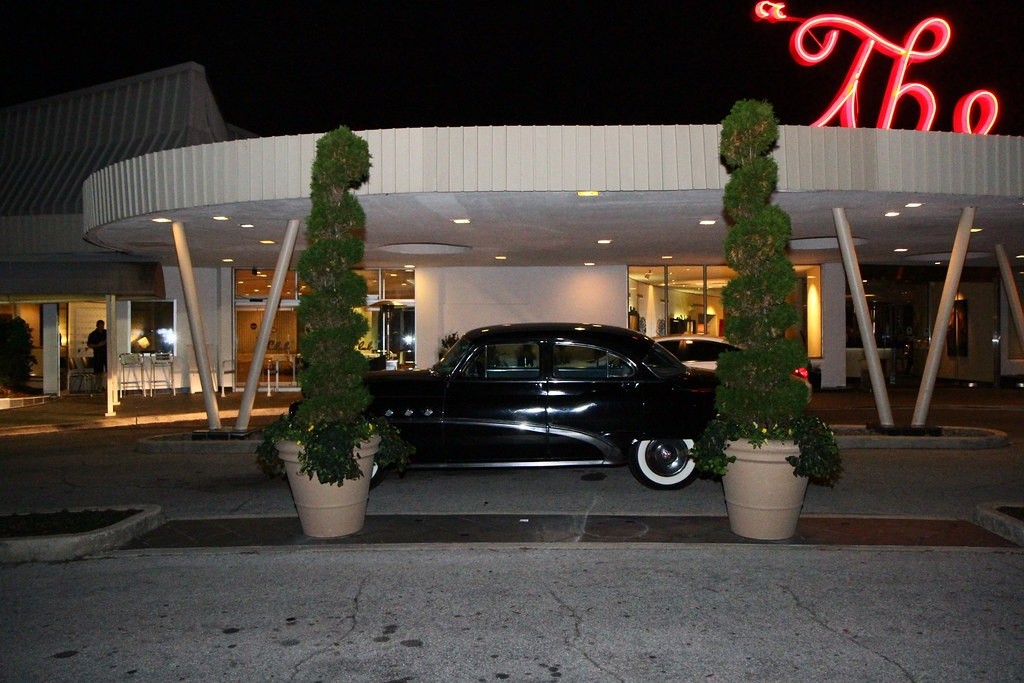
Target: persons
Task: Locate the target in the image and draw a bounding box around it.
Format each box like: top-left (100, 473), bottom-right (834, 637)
top-left (88, 319), bottom-right (106, 393)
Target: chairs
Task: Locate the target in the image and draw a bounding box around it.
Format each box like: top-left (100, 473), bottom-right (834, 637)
top-left (73, 357), bottom-right (99, 392)
top-left (69, 357), bottom-right (93, 394)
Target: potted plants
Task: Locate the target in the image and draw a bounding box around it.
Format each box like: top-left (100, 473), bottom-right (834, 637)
top-left (686, 99), bottom-right (846, 541)
top-left (254, 124), bottom-right (417, 537)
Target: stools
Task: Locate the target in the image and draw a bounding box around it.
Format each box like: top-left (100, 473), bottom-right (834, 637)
top-left (117, 354), bottom-right (146, 399)
top-left (150, 352), bottom-right (176, 399)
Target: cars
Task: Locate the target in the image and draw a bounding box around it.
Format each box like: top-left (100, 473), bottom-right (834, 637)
top-left (289, 321), bottom-right (722, 492)
top-left (652, 335), bottom-right (814, 406)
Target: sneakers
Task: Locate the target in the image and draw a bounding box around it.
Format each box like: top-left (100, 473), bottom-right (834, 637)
top-left (96, 388), bottom-right (102, 393)
